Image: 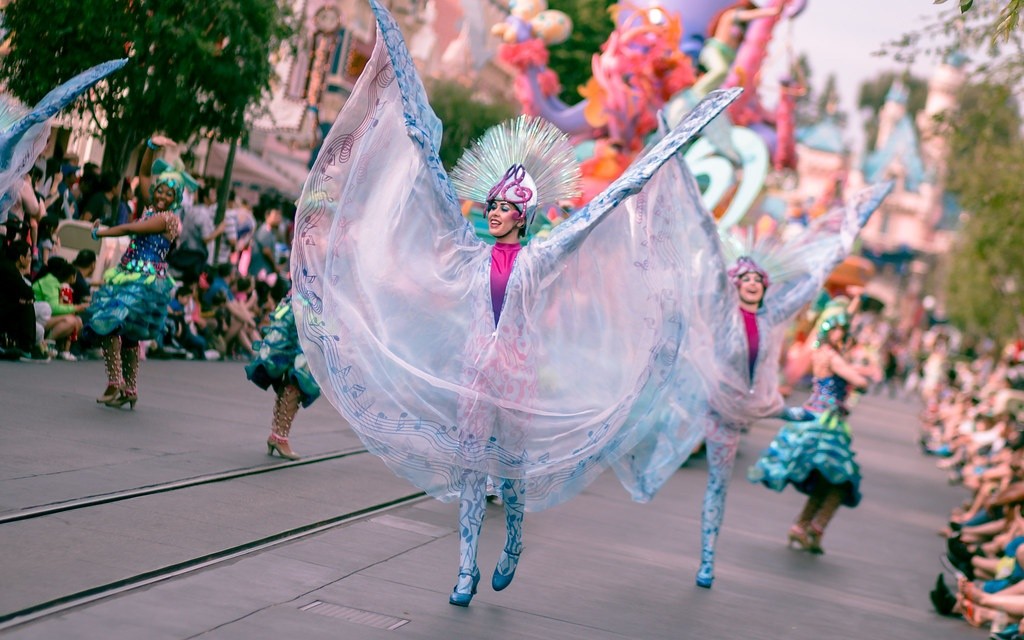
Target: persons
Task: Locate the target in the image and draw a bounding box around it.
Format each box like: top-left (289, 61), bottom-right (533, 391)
top-left (0, 118), bottom-right (299, 366)
top-left (745, 295), bottom-right (869, 555)
top-left (85, 136), bottom-right (202, 411)
top-left (694, 215), bottom-right (844, 590)
top-left (243, 164), bottom-right (347, 463)
top-left (915, 321), bottom-right (1023, 639)
top-left (786, 278), bottom-right (940, 396)
top-left (407, 121), bottom-right (647, 609)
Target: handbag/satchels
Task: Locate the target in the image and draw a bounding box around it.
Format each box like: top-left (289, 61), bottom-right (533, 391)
top-left (167, 222), bottom-right (208, 271)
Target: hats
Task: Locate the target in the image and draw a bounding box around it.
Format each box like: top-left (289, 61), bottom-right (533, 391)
top-left (151, 158), bottom-right (199, 210)
top-left (811, 292), bottom-right (850, 348)
top-left (61, 164), bottom-right (81, 174)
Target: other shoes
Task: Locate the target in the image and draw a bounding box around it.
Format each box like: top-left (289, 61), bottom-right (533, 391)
top-left (0, 344), bottom-right (103, 363)
top-left (186, 352), bottom-right (194, 360)
top-left (449, 569), bottom-right (480, 607)
top-left (205, 350), bottom-right (221, 360)
top-left (936, 574), bottom-right (950, 595)
top-left (989, 623), bottom-right (1019, 639)
top-left (946, 521), bottom-right (982, 581)
top-left (696, 569), bottom-right (714, 588)
top-left (492, 549), bottom-right (522, 591)
top-left (930, 590), bottom-right (961, 618)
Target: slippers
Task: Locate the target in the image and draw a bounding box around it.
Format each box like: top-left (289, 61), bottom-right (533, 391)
top-left (957, 592), bottom-right (983, 627)
top-left (957, 575), bottom-right (974, 602)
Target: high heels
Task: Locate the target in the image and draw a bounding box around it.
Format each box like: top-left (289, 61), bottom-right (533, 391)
top-left (105, 394), bottom-right (138, 411)
top-left (267, 436), bottom-right (299, 464)
top-left (787, 526), bottom-right (809, 550)
top-left (96, 384), bottom-right (124, 403)
top-left (805, 526), bottom-right (824, 553)
